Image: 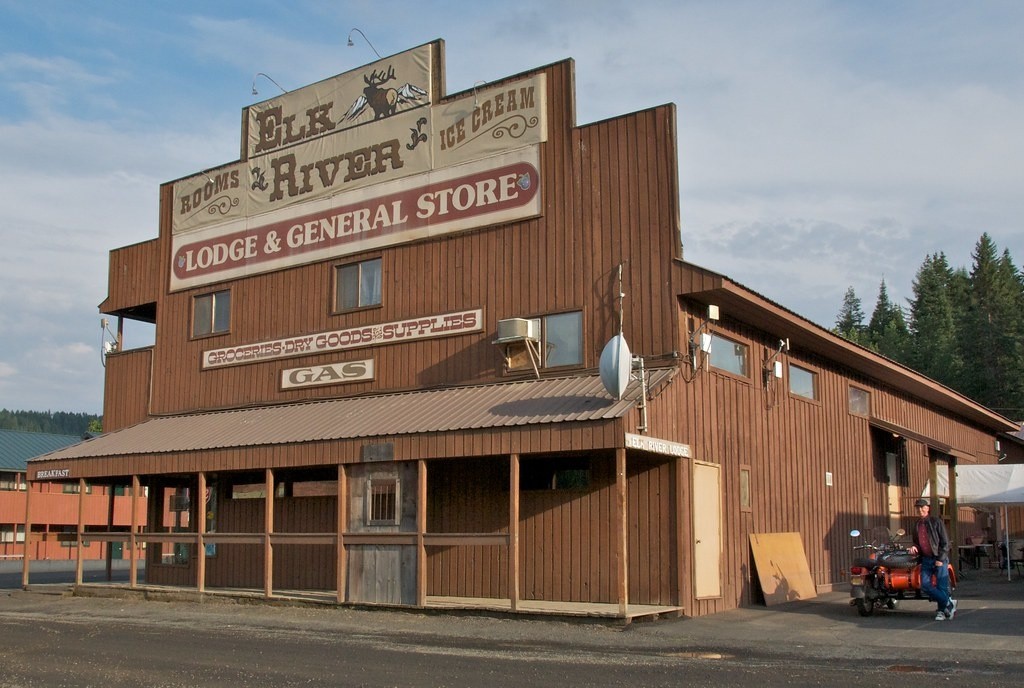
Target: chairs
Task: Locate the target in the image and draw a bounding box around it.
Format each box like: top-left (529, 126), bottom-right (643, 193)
top-left (1001, 539), bottom-right (1024, 578)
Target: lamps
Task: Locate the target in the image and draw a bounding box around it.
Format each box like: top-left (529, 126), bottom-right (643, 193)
top-left (201, 171), bottom-right (215, 183)
top-left (347, 28), bottom-right (382, 58)
top-left (474, 79), bottom-right (486, 108)
top-left (252, 73), bottom-right (287, 96)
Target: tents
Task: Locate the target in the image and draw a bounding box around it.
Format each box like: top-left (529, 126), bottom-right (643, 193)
top-left (920, 463), bottom-right (1024, 580)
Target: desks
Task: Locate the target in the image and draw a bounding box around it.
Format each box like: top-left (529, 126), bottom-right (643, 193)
top-left (957, 544), bottom-right (1001, 571)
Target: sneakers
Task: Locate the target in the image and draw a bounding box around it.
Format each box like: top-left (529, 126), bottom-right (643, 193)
top-left (946, 596), bottom-right (958, 620)
top-left (935, 609), bottom-right (946, 620)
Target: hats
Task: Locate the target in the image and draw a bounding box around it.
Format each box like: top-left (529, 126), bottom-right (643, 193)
top-left (914, 499), bottom-right (928, 506)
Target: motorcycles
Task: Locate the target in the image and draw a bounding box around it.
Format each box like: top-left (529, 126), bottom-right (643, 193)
top-left (839, 529), bottom-right (957, 616)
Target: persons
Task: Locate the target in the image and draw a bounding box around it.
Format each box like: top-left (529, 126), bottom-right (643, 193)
top-left (906, 499), bottom-right (958, 620)
